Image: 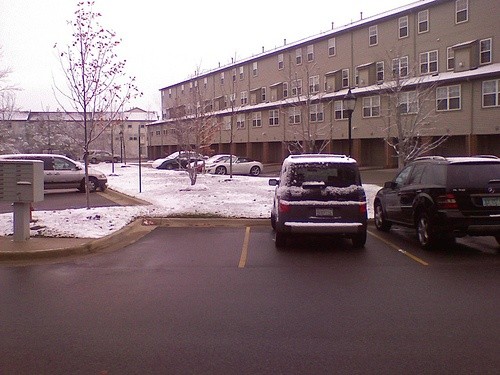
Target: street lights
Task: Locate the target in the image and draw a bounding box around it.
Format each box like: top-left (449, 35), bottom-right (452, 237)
top-left (117, 129), bottom-right (124, 164)
top-left (342, 87), bottom-right (357, 156)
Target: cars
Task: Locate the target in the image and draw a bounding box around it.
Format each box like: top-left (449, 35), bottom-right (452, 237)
top-left (187, 155), bottom-right (237, 176)
top-left (203, 157), bottom-right (263, 175)
top-left (152, 150), bottom-right (208, 170)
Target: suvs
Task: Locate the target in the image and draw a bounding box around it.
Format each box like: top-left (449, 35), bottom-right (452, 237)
top-left (269, 154), bottom-right (368, 250)
top-left (0, 154), bottom-right (107, 194)
top-left (373, 156), bottom-right (500, 247)
top-left (82, 150), bottom-right (120, 164)
